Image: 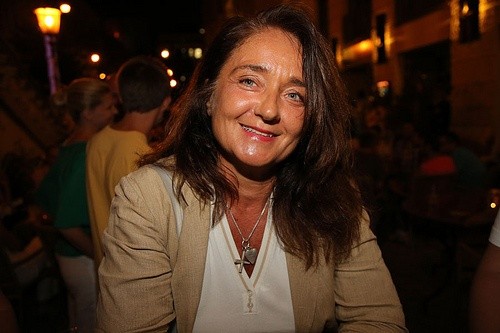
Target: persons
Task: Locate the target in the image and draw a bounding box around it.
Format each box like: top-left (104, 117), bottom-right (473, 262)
top-left (470, 206), bottom-right (500, 332)
top-left (84, 53), bottom-right (172, 299)
top-left (94, 1), bottom-right (409, 333)
top-left (30, 76), bottom-right (120, 333)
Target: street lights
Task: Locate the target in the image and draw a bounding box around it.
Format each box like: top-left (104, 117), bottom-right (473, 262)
top-left (89, 53), bottom-right (101, 66)
top-left (169, 79), bottom-right (177, 88)
top-left (34, 3), bottom-right (72, 100)
top-left (159, 48), bottom-right (171, 61)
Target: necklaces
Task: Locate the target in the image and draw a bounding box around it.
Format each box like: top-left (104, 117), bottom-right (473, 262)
top-left (222, 190), bottom-right (274, 273)
